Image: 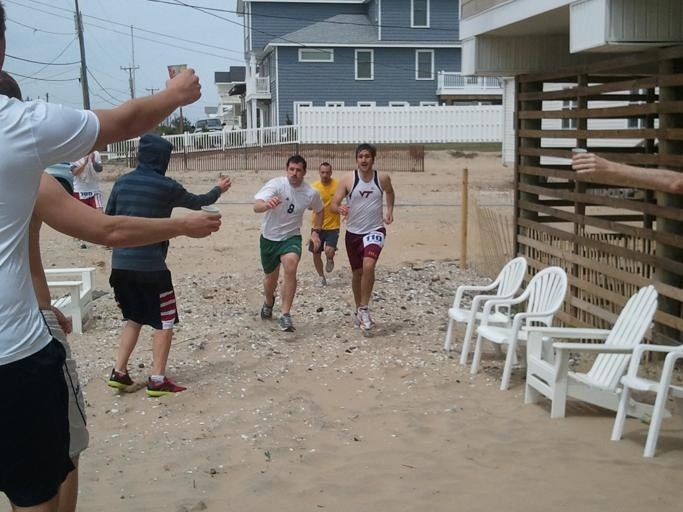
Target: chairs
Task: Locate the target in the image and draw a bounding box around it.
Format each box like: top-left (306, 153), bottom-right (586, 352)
top-left (609, 338), bottom-right (681, 466)
top-left (441, 256), bottom-right (528, 365)
top-left (470, 266), bottom-right (570, 391)
top-left (522, 282), bottom-right (676, 427)
top-left (45, 266), bottom-right (97, 334)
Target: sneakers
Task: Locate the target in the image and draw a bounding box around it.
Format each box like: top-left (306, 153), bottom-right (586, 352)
top-left (315, 275), bottom-right (326, 288)
top-left (325, 258), bottom-right (335, 273)
top-left (145, 375), bottom-right (187, 397)
top-left (277, 313), bottom-right (297, 333)
top-left (108, 367), bottom-right (138, 392)
top-left (354, 306), bottom-right (377, 330)
top-left (260, 296), bottom-right (275, 322)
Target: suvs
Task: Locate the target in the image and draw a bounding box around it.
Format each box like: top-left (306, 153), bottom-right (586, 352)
top-left (44, 162), bottom-right (73, 196)
top-left (188, 118), bottom-right (227, 149)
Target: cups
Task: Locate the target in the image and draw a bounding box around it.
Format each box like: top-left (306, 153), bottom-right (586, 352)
top-left (217, 175), bottom-right (228, 180)
top-left (571, 147), bottom-right (586, 159)
top-left (200, 204), bottom-right (219, 214)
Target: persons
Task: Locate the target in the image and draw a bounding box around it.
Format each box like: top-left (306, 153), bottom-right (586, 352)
top-left (1, 71), bottom-right (222, 512)
top-left (329, 143), bottom-right (395, 329)
top-left (69, 151), bottom-right (109, 249)
top-left (102, 135), bottom-right (232, 396)
top-left (1, 0), bottom-right (203, 512)
top-left (308, 162), bottom-right (341, 285)
top-left (253, 155), bottom-right (325, 333)
top-left (572, 153), bottom-right (683, 195)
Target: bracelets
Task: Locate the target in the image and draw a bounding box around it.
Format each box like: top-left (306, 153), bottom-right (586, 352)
top-left (313, 229), bottom-right (320, 233)
